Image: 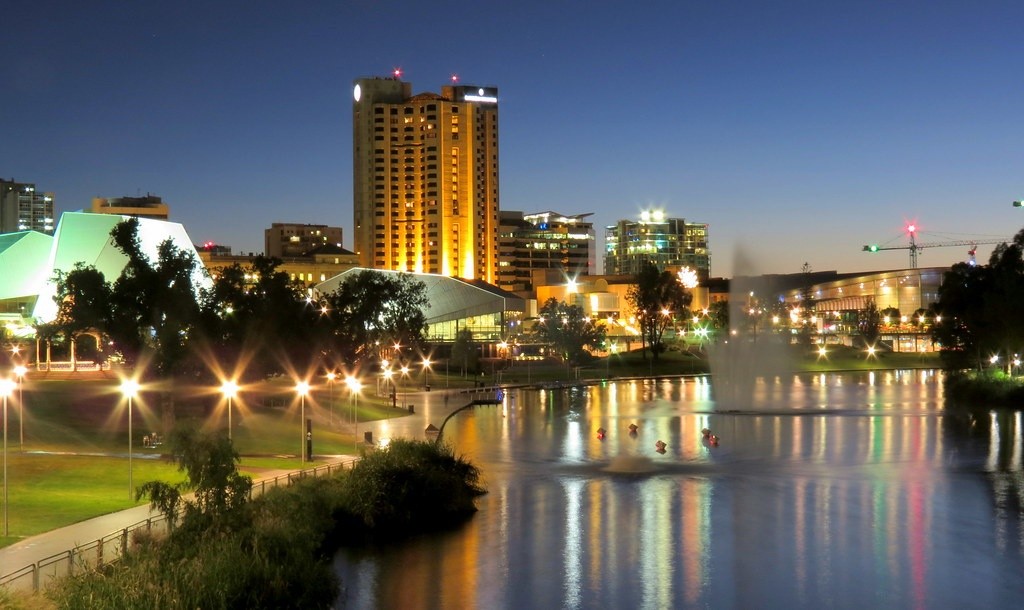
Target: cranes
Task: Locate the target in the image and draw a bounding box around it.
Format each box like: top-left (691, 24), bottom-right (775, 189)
top-left (862, 226), bottom-right (1016, 269)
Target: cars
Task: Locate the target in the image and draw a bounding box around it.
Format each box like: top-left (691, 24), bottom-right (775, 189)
top-left (968, 352), bottom-right (989, 368)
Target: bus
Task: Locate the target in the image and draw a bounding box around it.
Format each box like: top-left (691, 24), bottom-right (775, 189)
top-left (663, 310), bottom-right (719, 330)
top-left (815, 309), bottom-right (871, 325)
top-left (499, 344), bottom-right (549, 358)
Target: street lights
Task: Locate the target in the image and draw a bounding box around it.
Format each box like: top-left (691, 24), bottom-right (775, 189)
top-left (750, 310), bottom-right (762, 343)
top-left (424, 361), bottom-right (427, 385)
top-left (386, 371), bottom-right (390, 417)
top-left (347, 378), bottom-right (353, 424)
top-left (328, 374), bottom-right (334, 422)
top-left (353, 385), bottom-right (359, 450)
top-left (446, 359), bottom-right (449, 387)
top-left (0, 381), bottom-right (12, 536)
top-left (403, 368), bottom-right (407, 408)
top-left (15, 367), bottom-right (25, 450)
top-left (124, 382), bottom-right (134, 500)
top-left (299, 384), bottom-right (305, 463)
top-left (225, 384), bottom-right (234, 445)
